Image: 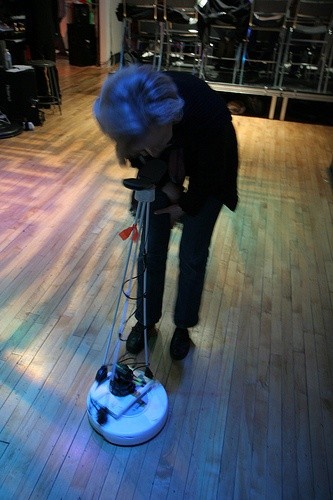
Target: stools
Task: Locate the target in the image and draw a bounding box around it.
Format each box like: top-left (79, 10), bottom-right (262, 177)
top-left (27, 60), bottom-right (62, 115)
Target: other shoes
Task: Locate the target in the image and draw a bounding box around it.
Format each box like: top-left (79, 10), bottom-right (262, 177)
top-left (170, 328), bottom-right (195, 361)
top-left (126, 322), bottom-right (155, 355)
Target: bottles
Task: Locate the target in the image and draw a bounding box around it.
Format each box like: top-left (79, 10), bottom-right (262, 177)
top-left (4, 48), bottom-right (12, 70)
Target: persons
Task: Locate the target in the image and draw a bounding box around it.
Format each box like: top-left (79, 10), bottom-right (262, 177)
top-left (23, 0), bottom-right (62, 106)
top-left (94, 67), bottom-right (239, 363)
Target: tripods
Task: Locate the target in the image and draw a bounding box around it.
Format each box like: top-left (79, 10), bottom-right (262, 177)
top-left (96, 188), bottom-right (156, 422)
